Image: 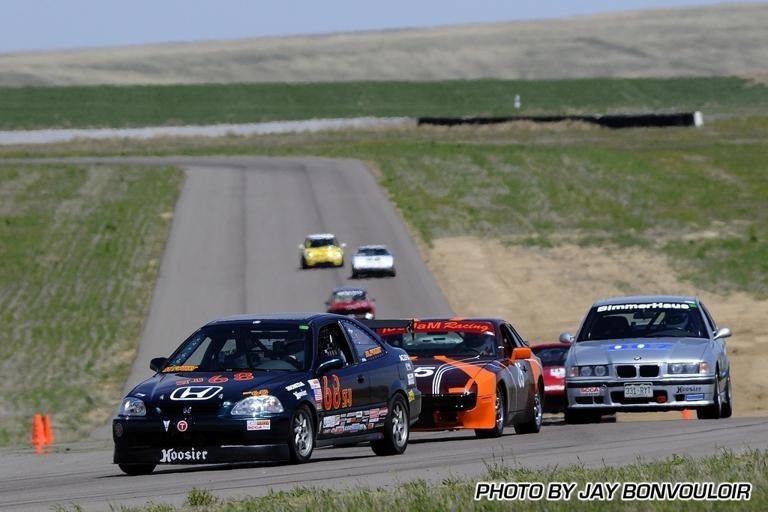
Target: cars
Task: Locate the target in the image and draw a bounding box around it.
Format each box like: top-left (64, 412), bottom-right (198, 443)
top-left (527, 343), bottom-right (614, 423)
top-left (388, 317), bottom-right (543, 438)
top-left (351, 244), bottom-right (395, 279)
top-left (322, 288), bottom-right (377, 322)
top-left (297, 233), bottom-right (347, 270)
top-left (561, 294), bottom-right (732, 423)
top-left (111, 316), bottom-right (426, 477)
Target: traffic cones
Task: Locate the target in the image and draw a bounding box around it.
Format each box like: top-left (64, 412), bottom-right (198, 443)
top-left (33, 414), bottom-right (52, 446)
